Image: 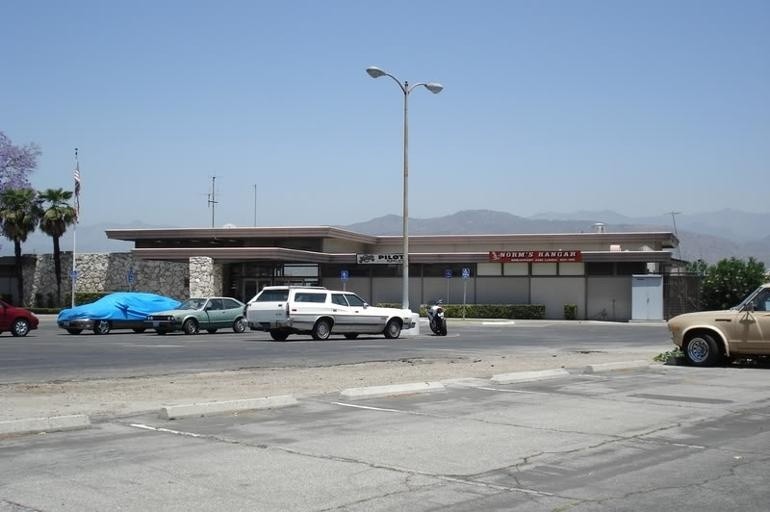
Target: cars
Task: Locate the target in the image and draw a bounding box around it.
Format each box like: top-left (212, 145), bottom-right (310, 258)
top-left (667, 283), bottom-right (770, 366)
top-left (0, 300), bottom-right (39, 337)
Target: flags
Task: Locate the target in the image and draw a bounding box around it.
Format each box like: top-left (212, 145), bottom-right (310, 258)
top-left (73, 152), bottom-right (80, 224)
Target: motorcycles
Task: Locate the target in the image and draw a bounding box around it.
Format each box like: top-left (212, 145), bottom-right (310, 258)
top-left (420, 297), bottom-right (447, 336)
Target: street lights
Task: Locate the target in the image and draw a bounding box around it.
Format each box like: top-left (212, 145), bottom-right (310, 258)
top-left (366, 66), bottom-right (443, 309)
